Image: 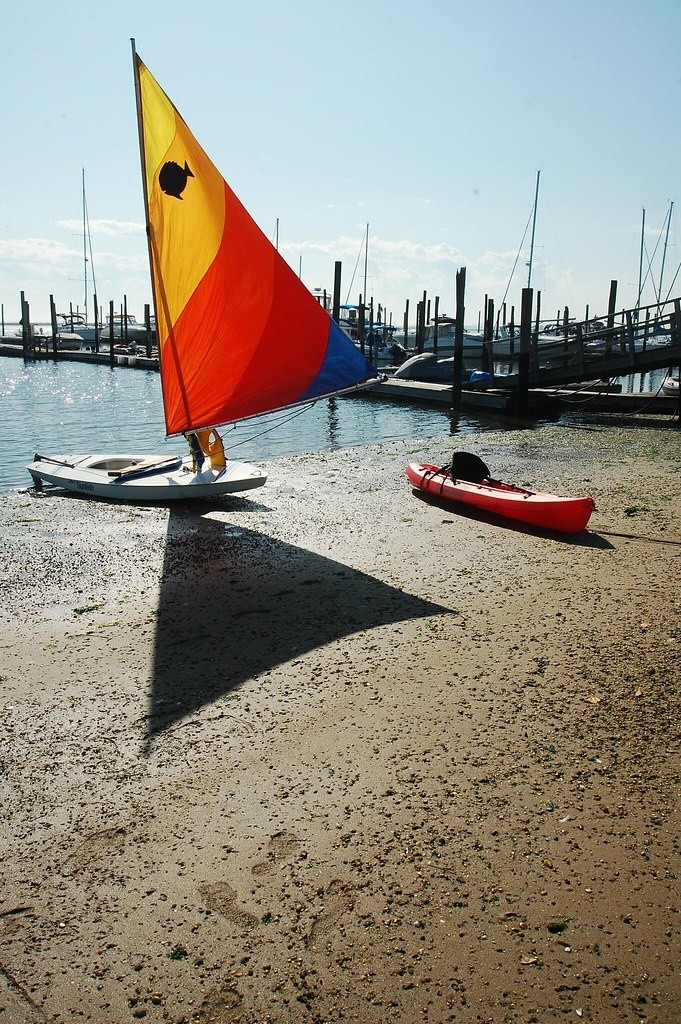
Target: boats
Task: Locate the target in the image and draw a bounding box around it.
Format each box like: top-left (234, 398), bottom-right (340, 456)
top-left (404, 462), bottom-right (599, 538)
top-left (0, 167), bottom-right (160, 359)
top-left (661, 376), bottom-right (680, 396)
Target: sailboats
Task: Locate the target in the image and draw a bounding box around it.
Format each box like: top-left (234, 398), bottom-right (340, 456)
top-left (338, 170), bottom-right (670, 367)
top-left (25, 33), bottom-right (391, 505)
